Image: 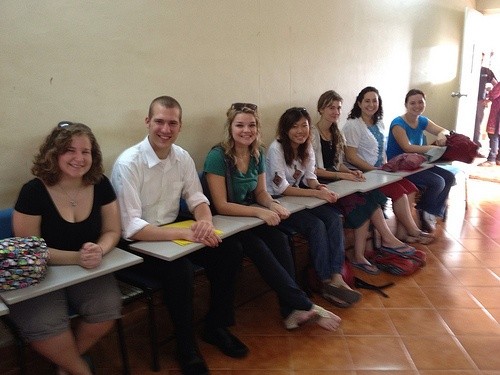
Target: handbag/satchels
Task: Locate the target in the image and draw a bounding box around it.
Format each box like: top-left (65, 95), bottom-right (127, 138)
top-left (0, 236), bottom-right (50, 292)
top-left (383, 152), bottom-right (426, 173)
top-left (368, 247), bottom-right (427, 277)
top-left (426, 132), bottom-right (480, 164)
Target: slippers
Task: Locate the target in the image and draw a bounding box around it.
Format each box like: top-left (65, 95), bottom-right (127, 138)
top-left (349, 258), bottom-right (380, 275)
top-left (380, 242), bottom-right (417, 258)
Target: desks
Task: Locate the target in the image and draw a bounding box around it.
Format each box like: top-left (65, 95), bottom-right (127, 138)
top-left (0, 157), bottom-right (472, 317)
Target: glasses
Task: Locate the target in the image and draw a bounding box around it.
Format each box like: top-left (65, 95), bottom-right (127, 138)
top-left (286, 107), bottom-right (307, 113)
top-left (231, 103), bottom-right (257, 113)
top-left (55, 121), bottom-right (72, 144)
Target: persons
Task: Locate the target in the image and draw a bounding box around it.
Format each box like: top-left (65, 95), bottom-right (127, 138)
top-left (473, 52), bottom-right (500, 167)
top-left (109, 96), bottom-right (251, 375)
top-left (386, 89), bottom-right (455, 232)
top-left (201, 102), bottom-right (342, 332)
top-left (262, 107), bottom-right (363, 307)
top-left (344, 88), bottom-right (438, 244)
top-left (9, 120), bottom-right (121, 375)
top-left (312, 90), bottom-right (417, 275)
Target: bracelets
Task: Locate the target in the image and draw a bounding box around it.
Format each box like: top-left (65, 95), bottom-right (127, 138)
top-left (272, 199), bottom-right (280, 204)
top-left (100, 247), bottom-right (103, 257)
top-left (316, 184), bottom-right (327, 190)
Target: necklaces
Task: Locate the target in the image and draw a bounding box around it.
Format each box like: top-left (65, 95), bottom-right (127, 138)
top-left (242, 168), bottom-right (247, 172)
top-left (70, 200), bottom-right (80, 206)
top-left (317, 122), bottom-right (328, 140)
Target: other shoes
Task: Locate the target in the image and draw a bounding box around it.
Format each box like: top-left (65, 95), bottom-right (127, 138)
top-left (284, 304), bottom-right (331, 328)
top-left (478, 160), bottom-right (500, 167)
top-left (418, 208), bottom-right (436, 232)
top-left (179, 335), bottom-right (210, 375)
top-left (201, 323), bottom-right (250, 358)
top-left (317, 283), bottom-right (360, 308)
top-left (404, 231), bottom-right (436, 245)
